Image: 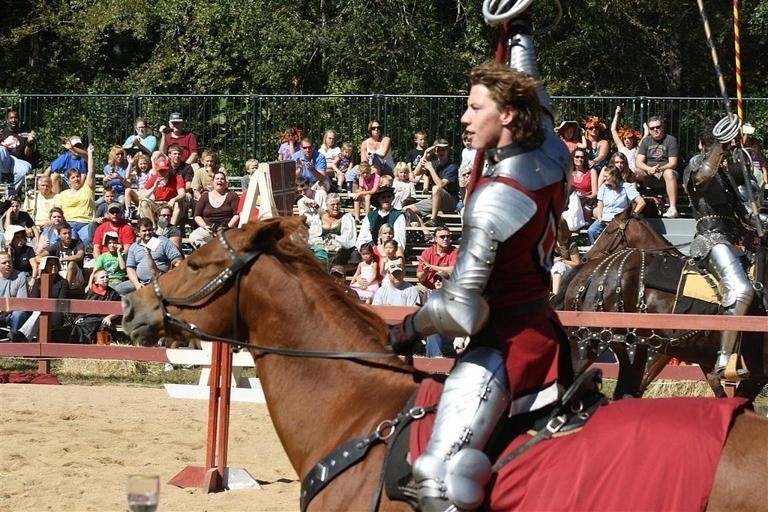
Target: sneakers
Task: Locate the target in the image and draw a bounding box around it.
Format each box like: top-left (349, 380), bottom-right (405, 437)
top-left (6, 329), bottom-right (27, 344)
top-left (718, 366), bottom-right (751, 378)
top-left (660, 208), bottom-right (679, 218)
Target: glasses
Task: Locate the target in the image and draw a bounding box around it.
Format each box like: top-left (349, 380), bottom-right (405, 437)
top-left (573, 155), bottom-right (586, 159)
top-left (648, 125), bottom-right (663, 131)
top-left (434, 234), bottom-right (451, 239)
top-left (390, 263), bottom-right (403, 269)
top-left (157, 213), bottom-right (172, 219)
top-left (370, 126), bottom-right (382, 130)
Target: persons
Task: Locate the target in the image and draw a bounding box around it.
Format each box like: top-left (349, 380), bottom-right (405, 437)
top-left (389, 1), bottom-right (574, 512)
top-left (682, 127), bottom-right (768, 382)
top-left (748, 137), bottom-right (768, 185)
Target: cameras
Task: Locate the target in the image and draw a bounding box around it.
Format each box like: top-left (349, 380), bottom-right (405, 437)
top-left (211, 223), bottom-right (218, 231)
top-left (162, 128), bottom-right (174, 133)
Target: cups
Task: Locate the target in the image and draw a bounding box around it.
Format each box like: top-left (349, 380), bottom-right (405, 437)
top-left (126, 474), bottom-right (160, 512)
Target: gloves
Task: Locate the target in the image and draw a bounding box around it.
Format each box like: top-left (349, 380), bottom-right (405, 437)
top-left (387, 310), bottom-right (424, 357)
top-left (506, 0), bottom-right (533, 34)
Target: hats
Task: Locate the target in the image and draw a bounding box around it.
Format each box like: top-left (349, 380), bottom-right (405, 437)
top-left (168, 112), bottom-right (185, 122)
top-left (432, 271), bottom-right (450, 280)
top-left (70, 136), bottom-right (82, 146)
top-left (154, 158), bottom-right (170, 171)
top-left (369, 186), bottom-right (396, 209)
top-left (386, 261), bottom-right (404, 273)
top-left (107, 202), bottom-right (123, 212)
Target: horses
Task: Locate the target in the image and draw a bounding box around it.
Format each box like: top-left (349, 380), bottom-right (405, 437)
top-left (580, 194), bottom-right (728, 399)
top-left (552, 247), bottom-right (767, 411)
top-left (120, 216), bottom-right (768, 512)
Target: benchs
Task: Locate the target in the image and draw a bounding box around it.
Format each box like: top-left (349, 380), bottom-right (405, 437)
top-left (228, 176), bottom-right (243, 196)
top-left (402, 212), bottom-right (462, 287)
top-left (292, 191), bottom-right (372, 232)
top-left (95, 174), bottom-right (106, 197)
